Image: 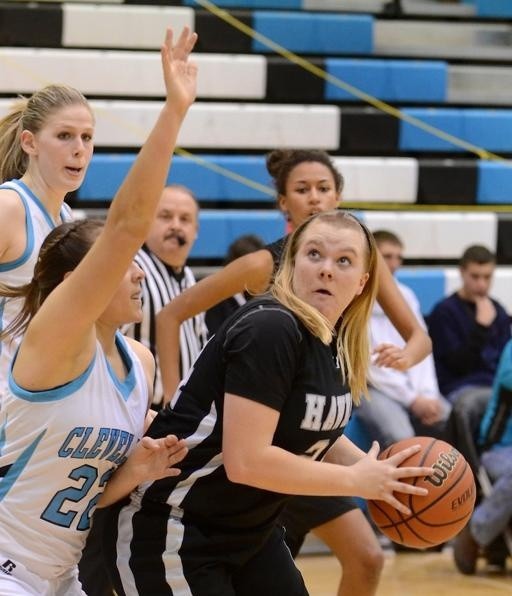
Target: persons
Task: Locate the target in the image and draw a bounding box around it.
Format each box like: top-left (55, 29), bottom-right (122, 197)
top-left (454, 338), bottom-right (511, 574)
top-left (206, 238), bottom-right (265, 332)
top-left (102, 211), bottom-right (433, 595)
top-left (156, 149), bottom-right (433, 594)
top-left (423, 246), bottom-right (511, 429)
top-left (119, 184), bottom-right (208, 435)
top-left (348, 231), bottom-right (453, 450)
top-left (0, 83), bottom-right (95, 393)
top-left (0, 24), bottom-right (199, 596)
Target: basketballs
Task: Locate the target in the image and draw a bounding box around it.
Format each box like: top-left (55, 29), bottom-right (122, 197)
top-left (367, 437), bottom-right (477, 548)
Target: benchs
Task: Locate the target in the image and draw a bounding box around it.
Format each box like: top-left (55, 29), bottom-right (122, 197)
top-left (2, 1), bottom-right (511, 556)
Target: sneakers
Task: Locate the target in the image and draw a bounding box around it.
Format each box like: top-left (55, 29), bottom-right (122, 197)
top-left (454, 523), bottom-right (479, 574)
